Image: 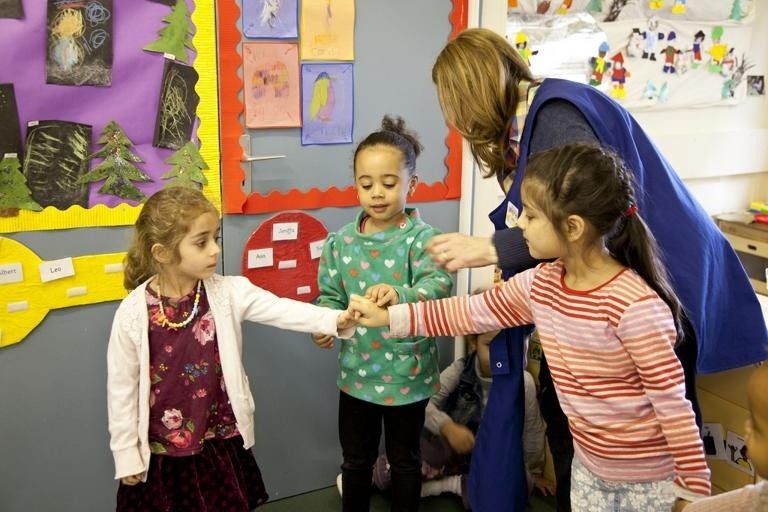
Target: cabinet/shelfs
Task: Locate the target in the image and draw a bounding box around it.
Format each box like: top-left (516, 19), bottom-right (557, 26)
top-left (717, 215), bottom-right (768, 296)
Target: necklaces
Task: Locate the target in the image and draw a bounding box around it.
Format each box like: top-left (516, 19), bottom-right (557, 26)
top-left (153, 280), bottom-right (202, 329)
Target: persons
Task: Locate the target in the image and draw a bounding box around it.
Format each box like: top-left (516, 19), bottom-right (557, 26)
top-left (680, 358), bottom-right (767, 512)
top-left (308, 115), bottom-right (456, 511)
top-left (347, 146), bottom-right (712, 512)
top-left (101, 188), bottom-right (362, 510)
top-left (334, 286), bottom-right (557, 498)
top-left (426, 27), bottom-right (767, 512)
